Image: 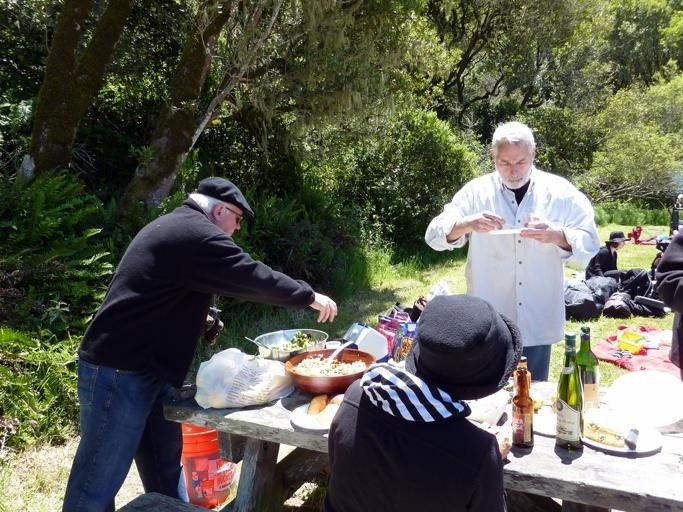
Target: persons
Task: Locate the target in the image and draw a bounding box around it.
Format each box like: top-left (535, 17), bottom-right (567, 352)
top-left (583, 230), bottom-right (632, 280)
top-left (653, 226), bottom-right (683, 383)
top-left (424, 123), bottom-right (602, 382)
top-left (61, 176), bottom-right (339, 511)
top-left (628, 225), bottom-right (643, 244)
top-left (670, 194), bottom-right (682, 238)
top-left (323, 293), bottom-right (522, 511)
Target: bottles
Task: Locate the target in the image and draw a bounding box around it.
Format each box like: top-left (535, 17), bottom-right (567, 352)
top-left (556, 331), bottom-right (583, 449)
top-left (511, 370), bottom-right (533, 453)
top-left (575, 327), bottom-right (601, 410)
top-left (514, 357), bottom-right (532, 394)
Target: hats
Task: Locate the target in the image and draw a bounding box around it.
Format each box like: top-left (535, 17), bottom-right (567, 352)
top-left (605, 230), bottom-right (631, 243)
top-left (196, 176), bottom-right (254, 220)
top-left (403, 293), bottom-right (524, 402)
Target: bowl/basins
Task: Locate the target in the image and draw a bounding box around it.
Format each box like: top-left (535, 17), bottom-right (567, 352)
top-left (285, 348), bottom-right (377, 395)
top-left (254, 328), bottom-right (329, 362)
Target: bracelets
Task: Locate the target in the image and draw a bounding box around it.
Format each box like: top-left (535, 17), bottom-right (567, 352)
top-left (553, 228), bottom-right (564, 243)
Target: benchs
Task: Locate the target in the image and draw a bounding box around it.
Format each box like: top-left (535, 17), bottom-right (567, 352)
top-left (116, 491), bottom-right (218, 512)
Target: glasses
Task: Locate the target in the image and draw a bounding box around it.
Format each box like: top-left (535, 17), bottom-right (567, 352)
top-left (226, 207), bottom-right (245, 224)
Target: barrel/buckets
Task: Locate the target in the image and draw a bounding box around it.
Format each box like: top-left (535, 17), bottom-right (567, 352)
top-left (177, 423), bottom-right (234, 508)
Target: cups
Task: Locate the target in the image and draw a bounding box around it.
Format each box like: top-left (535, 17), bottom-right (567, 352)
top-left (326, 341), bottom-right (341, 349)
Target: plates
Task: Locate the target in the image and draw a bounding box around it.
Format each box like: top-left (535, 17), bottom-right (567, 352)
top-left (608, 369), bottom-right (682, 427)
top-left (488, 226), bottom-right (534, 236)
top-left (582, 424), bottom-right (664, 456)
top-left (292, 402), bottom-right (341, 431)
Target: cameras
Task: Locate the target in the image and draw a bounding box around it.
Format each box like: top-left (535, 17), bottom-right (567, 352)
top-left (203, 307), bottom-right (226, 345)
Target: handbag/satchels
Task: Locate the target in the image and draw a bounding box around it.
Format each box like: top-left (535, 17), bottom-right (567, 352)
top-left (378, 305), bottom-right (411, 327)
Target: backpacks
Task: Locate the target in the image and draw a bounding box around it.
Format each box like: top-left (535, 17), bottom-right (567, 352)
top-left (565, 268), bottom-right (666, 318)
